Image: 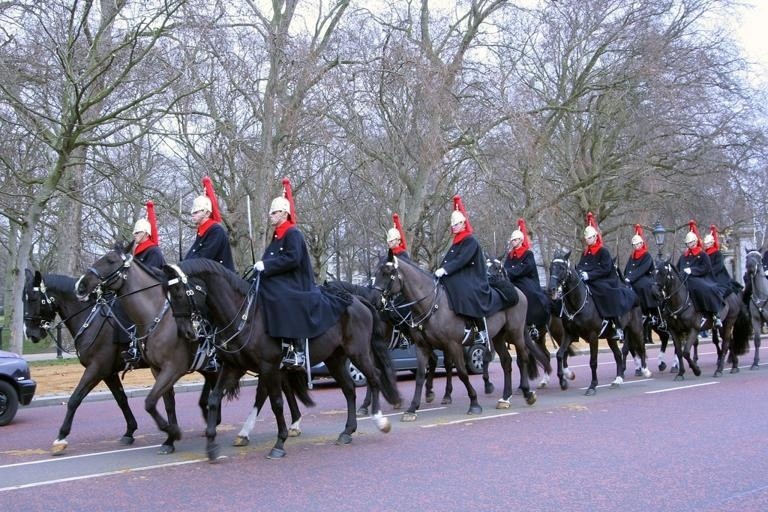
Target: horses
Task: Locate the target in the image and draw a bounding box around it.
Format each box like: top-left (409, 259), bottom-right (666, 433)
top-left (161, 258), bottom-right (403, 463)
top-left (21, 268), bottom-right (221, 454)
top-left (323, 247), bottom-right (768, 422)
top-left (73, 234), bottom-right (316, 454)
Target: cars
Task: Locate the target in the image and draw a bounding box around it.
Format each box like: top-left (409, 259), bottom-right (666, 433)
top-left (1, 351), bottom-right (36, 427)
top-left (303, 329), bottom-right (494, 387)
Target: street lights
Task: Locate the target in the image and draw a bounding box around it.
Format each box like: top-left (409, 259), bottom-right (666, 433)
top-left (651, 218), bottom-right (666, 260)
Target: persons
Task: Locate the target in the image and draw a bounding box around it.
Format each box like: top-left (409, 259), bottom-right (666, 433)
top-left (675, 220), bottom-right (727, 329)
top-left (114, 201), bottom-right (167, 369)
top-left (624, 224), bottom-right (660, 328)
top-left (703, 224), bottom-right (744, 300)
top-left (435, 195), bottom-right (509, 345)
top-left (574, 211), bottom-right (636, 340)
top-left (366, 213), bottom-right (410, 349)
top-left (253, 177), bottom-right (353, 371)
top-left (743, 249), bottom-right (768, 306)
top-left (183, 176), bottom-right (236, 372)
top-left (504, 218), bottom-right (551, 344)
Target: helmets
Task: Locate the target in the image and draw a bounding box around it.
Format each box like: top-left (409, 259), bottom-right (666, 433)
top-left (584, 212), bottom-right (603, 244)
top-left (684, 220), bottom-right (703, 249)
top-left (631, 224), bottom-right (647, 250)
top-left (510, 218), bottom-right (529, 249)
top-left (387, 213), bottom-right (406, 249)
top-left (451, 194), bottom-right (471, 232)
top-left (132, 194), bottom-right (291, 235)
top-left (703, 224), bottom-right (719, 250)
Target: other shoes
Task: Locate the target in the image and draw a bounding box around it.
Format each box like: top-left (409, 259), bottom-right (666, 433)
top-left (648, 316), bottom-right (660, 325)
top-left (611, 328), bottom-right (624, 339)
top-left (658, 324), bottom-right (667, 331)
top-left (128, 348), bottom-right (141, 360)
top-left (531, 328), bottom-right (540, 340)
top-left (203, 357), bottom-right (221, 371)
top-left (475, 329), bottom-right (487, 342)
top-left (714, 318), bottom-right (723, 328)
top-left (282, 345), bottom-right (306, 372)
